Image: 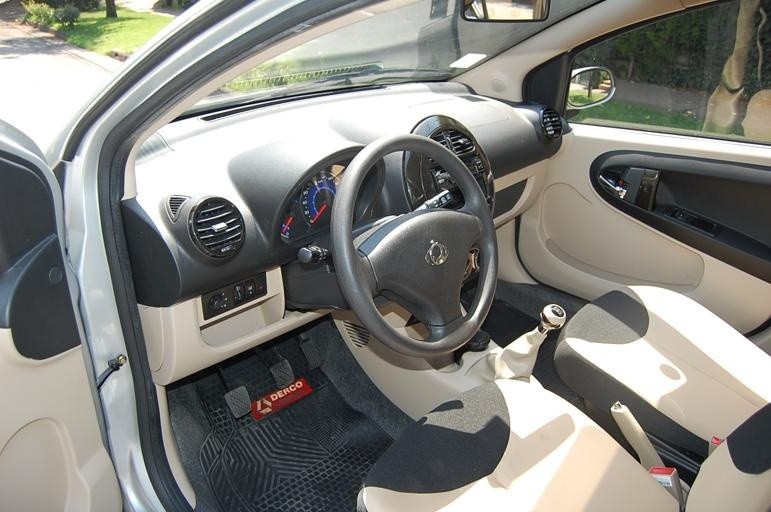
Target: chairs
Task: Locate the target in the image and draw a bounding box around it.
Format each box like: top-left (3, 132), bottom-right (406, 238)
top-left (356, 377), bottom-right (771, 511)
top-left (552, 280), bottom-right (771, 485)
